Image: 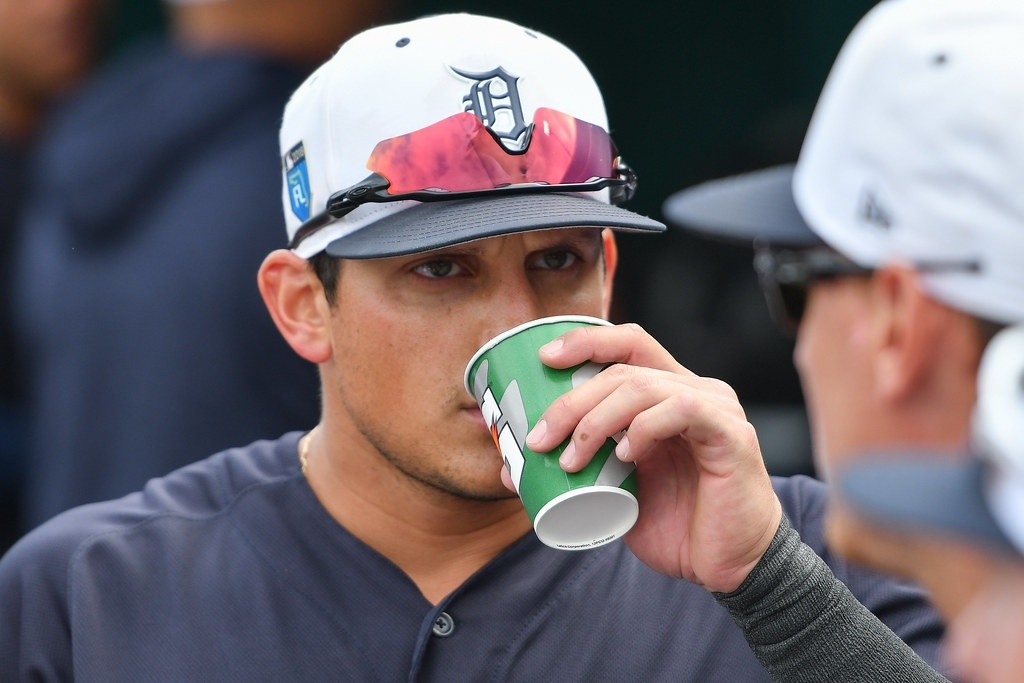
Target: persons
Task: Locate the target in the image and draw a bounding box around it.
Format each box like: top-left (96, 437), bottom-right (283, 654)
top-left (660, 2), bottom-right (1023, 681)
top-left (2, 0), bottom-right (402, 549)
top-left (2, 12), bottom-right (951, 683)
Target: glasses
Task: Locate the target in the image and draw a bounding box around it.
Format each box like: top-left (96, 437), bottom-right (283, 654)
top-left (750, 239), bottom-right (983, 330)
top-left (282, 107), bottom-right (637, 249)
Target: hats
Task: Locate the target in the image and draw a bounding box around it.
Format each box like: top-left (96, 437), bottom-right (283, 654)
top-left (278, 10), bottom-right (667, 261)
top-left (838, 325), bottom-right (1024, 562)
top-left (661, 1), bottom-right (1024, 326)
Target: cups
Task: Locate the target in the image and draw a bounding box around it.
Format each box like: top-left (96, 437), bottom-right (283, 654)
top-left (465, 315), bottom-right (640, 551)
